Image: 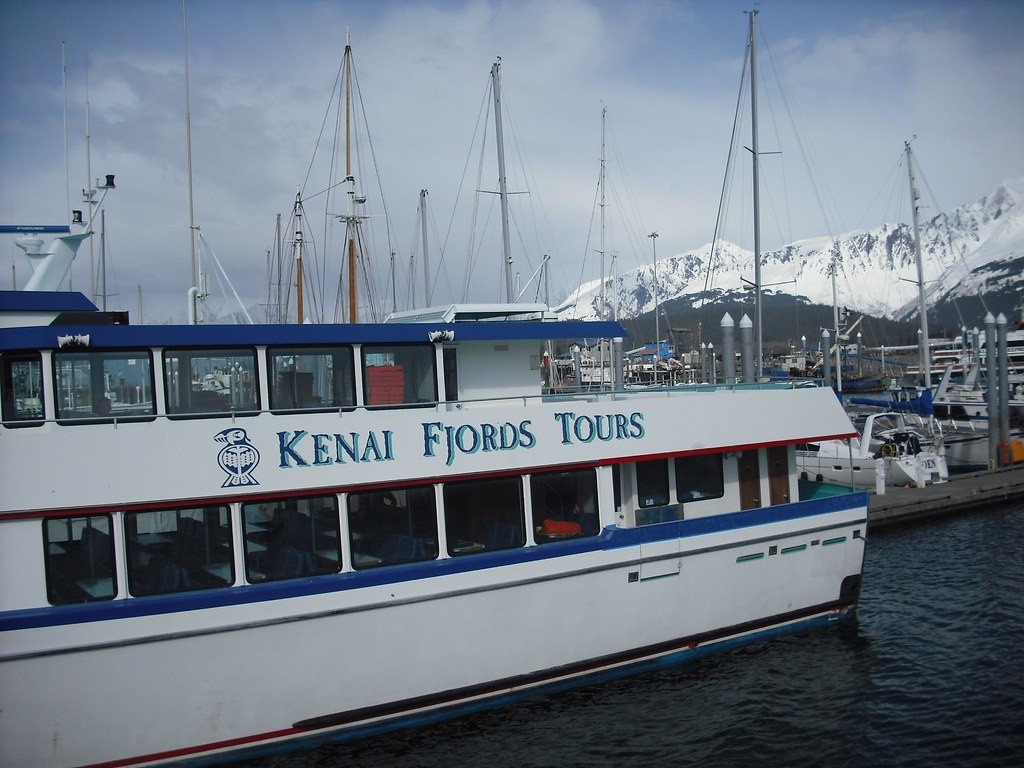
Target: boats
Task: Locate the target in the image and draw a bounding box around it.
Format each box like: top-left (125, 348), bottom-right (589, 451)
top-left (0, 171), bottom-right (871, 768)
top-left (767, 132), bottom-right (1024, 474)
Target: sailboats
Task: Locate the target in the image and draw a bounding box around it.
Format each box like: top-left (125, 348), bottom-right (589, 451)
top-left (0, 0), bottom-right (699, 422)
top-left (691, 0), bottom-right (954, 487)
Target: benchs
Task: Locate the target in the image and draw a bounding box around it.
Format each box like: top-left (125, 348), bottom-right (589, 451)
top-left (52, 509), bottom-right (523, 605)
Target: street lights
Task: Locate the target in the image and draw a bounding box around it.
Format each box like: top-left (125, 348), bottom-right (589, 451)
top-left (647, 231), bottom-right (662, 362)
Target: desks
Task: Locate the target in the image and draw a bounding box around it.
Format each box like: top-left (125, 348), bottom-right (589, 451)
top-left (47, 542), bottom-right (67, 560)
top-left (319, 531), bottom-right (362, 541)
top-left (128, 533), bottom-right (174, 545)
top-left (222, 524), bottom-right (268, 536)
top-left (424, 538), bottom-right (484, 552)
top-left (313, 549), bottom-right (383, 568)
top-left (222, 539), bottom-right (267, 555)
top-left (200, 562), bottom-right (265, 583)
top-left (72, 577), bottom-right (115, 599)
